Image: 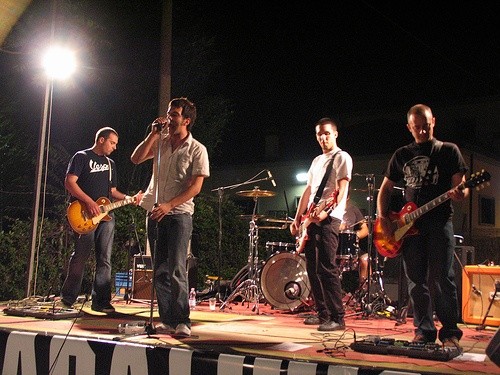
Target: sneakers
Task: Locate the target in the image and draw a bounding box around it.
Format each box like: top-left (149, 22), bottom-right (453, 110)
top-left (175, 323), bottom-right (192, 338)
top-left (154, 324), bottom-right (175, 333)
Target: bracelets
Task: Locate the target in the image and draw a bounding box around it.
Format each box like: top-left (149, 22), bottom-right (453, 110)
top-left (124, 195), bottom-right (127, 199)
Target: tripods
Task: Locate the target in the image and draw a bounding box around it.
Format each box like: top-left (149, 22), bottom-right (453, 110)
top-left (219, 194), bottom-right (261, 315)
top-left (344, 172), bottom-right (403, 323)
top-left (113, 125), bottom-right (200, 341)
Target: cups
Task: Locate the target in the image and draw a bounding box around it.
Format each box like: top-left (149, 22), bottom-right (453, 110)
top-left (209, 298), bottom-right (216, 310)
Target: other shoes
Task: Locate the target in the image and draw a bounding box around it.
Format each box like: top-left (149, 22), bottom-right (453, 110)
top-left (303, 317), bottom-right (329, 325)
top-left (60, 300), bottom-right (72, 309)
top-left (442, 335), bottom-right (462, 349)
top-left (412, 336), bottom-right (436, 345)
top-left (317, 320), bottom-right (345, 331)
top-left (92, 303), bottom-right (115, 313)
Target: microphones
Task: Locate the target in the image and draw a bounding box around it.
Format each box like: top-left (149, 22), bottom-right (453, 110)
top-left (266, 170), bottom-right (276, 186)
top-left (151, 119), bottom-right (170, 125)
top-left (472, 284), bottom-right (482, 296)
top-left (289, 288), bottom-right (295, 294)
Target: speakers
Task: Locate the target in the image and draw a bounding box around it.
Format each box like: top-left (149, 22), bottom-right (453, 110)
top-left (486, 328), bottom-right (500, 368)
top-left (452, 246), bottom-right (500, 327)
top-left (132, 254), bottom-right (158, 300)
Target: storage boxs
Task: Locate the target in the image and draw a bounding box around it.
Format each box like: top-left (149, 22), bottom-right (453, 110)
top-left (114, 273), bottom-right (133, 297)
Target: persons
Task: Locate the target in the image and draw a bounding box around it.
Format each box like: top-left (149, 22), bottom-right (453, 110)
top-left (52, 127), bottom-right (142, 312)
top-left (131, 98), bottom-right (210, 336)
top-left (378, 104), bottom-right (469, 350)
top-left (290, 119), bottom-right (353, 332)
top-left (339, 180), bottom-right (372, 292)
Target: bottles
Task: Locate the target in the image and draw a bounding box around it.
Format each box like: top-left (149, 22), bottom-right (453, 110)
top-left (190, 288), bottom-right (196, 307)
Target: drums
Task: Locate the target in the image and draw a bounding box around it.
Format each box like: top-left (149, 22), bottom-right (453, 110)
top-left (265, 242), bottom-right (295, 258)
top-left (335, 230), bottom-right (359, 259)
top-left (261, 252), bottom-right (311, 310)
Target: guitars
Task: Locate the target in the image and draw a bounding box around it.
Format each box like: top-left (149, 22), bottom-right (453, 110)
top-left (65, 193), bottom-right (145, 235)
top-left (374, 169), bottom-right (491, 258)
top-left (294, 190), bottom-right (339, 252)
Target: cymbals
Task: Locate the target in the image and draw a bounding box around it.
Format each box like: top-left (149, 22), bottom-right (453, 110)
top-left (235, 190), bottom-right (277, 197)
top-left (349, 187), bottom-right (380, 192)
top-left (261, 219), bottom-right (293, 223)
top-left (361, 220), bottom-right (375, 223)
top-left (236, 215), bottom-right (267, 220)
